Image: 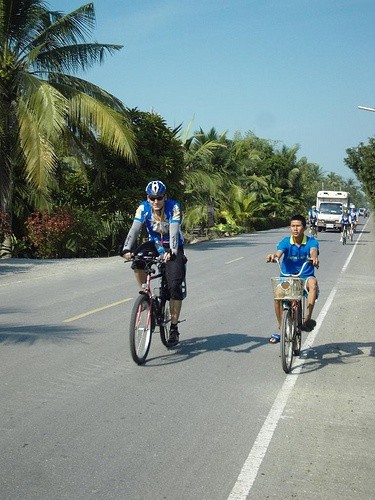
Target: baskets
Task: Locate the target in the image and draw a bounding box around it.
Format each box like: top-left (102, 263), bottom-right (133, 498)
top-left (271, 278), bottom-right (305, 300)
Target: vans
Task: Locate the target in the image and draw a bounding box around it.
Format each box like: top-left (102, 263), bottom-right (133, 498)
top-left (349, 203), bottom-right (366, 216)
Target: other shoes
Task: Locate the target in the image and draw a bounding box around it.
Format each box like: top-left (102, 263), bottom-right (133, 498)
top-left (315, 235), bottom-right (318, 238)
top-left (137, 301), bottom-right (146, 311)
top-left (168, 328), bottom-right (179, 345)
top-left (309, 231), bottom-right (312, 233)
top-left (340, 238), bottom-right (342, 241)
top-left (348, 234), bottom-right (351, 236)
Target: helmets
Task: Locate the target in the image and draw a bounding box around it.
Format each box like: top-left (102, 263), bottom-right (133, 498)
top-left (312, 206), bottom-right (316, 208)
top-left (145, 181), bottom-right (166, 196)
top-left (344, 209), bottom-right (347, 213)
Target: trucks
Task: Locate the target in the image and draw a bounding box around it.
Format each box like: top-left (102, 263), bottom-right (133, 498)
top-left (315, 191), bottom-right (350, 233)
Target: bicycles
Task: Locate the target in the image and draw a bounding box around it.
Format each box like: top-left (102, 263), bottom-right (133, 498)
top-left (342, 221), bottom-right (358, 245)
top-left (266, 256), bottom-right (320, 374)
top-left (124, 251), bottom-right (186, 365)
top-left (310, 219), bottom-right (318, 238)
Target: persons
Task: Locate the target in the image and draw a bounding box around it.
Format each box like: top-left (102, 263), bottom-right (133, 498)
top-left (120, 181), bottom-right (187, 346)
top-left (350, 208), bottom-right (358, 235)
top-left (266, 214), bottom-right (320, 344)
top-left (309, 205), bottom-right (320, 238)
top-left (339, 208), bottom-right (351, 243)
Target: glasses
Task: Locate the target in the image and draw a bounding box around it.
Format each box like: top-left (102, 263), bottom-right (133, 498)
top-left (149, 196), bottom-right (164, 201)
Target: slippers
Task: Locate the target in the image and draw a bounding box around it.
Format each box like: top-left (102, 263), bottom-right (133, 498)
top-left (304, 320), bottom-right (316, 332)
top-left (269, 334), bottom-right (280, 343)
